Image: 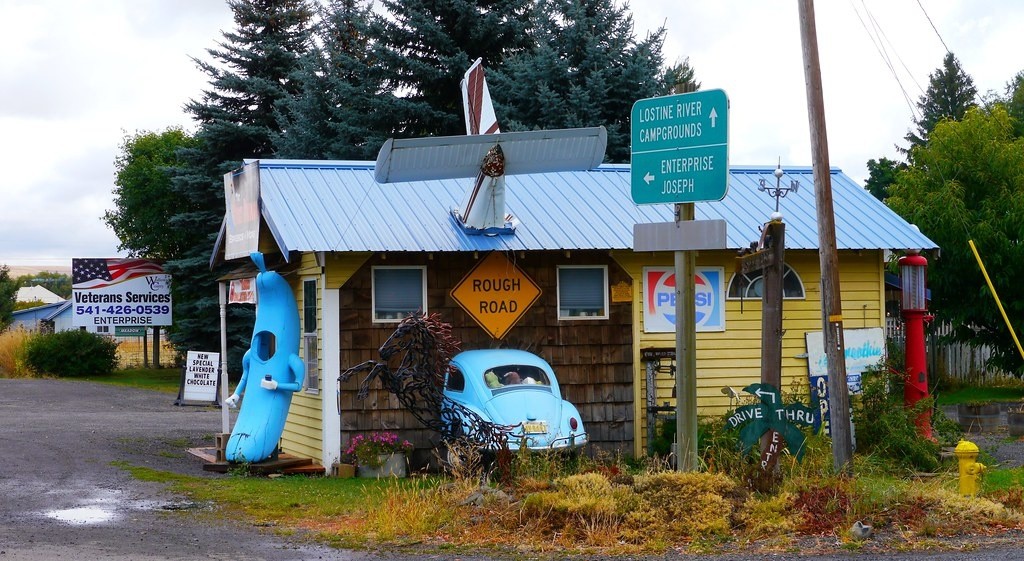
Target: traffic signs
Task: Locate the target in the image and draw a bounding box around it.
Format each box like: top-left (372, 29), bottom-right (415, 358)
top-left (630, 87), bottom-right (731, 204)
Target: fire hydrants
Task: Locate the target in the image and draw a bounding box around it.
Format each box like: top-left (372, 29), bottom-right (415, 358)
top-left (955, 438), bottom-right (988, 501)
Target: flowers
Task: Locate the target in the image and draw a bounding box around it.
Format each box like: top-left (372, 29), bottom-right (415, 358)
top-left (341, 428), bottom-right (413, 468)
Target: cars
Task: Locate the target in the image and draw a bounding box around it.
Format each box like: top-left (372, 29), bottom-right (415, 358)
top-left (442, 346), bottom-right (588, 466)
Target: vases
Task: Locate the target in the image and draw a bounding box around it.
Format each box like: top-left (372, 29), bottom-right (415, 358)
top-left (353, 450), bottom-right (407, 477)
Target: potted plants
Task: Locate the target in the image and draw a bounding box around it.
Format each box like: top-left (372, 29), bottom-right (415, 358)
top-left (1006, 398), bottom-right (1023, 436)
top-left (957, 400), bottom-right (1001, 433)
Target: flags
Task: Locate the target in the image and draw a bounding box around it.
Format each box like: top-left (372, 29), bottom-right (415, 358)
top-left (73, 258), bottom-right (170, 290)
